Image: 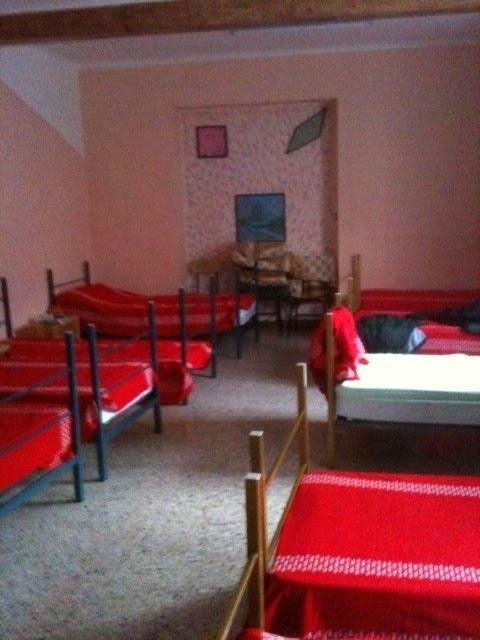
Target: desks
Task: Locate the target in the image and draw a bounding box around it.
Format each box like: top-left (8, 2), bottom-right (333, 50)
top-left (239, 284), bottom-right (288, 330)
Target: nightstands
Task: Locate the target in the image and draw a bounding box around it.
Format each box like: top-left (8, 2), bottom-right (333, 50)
top-left (16, 314), bottom-right (80, 342)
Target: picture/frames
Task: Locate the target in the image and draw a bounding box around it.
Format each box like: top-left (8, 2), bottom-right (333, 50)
top-left (197, 126), bottom-right (229, 158)
top-left (235, 193), bottom-right (286, 242)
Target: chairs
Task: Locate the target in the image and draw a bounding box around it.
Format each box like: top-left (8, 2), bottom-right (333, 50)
top-left (190, 258), bottom-right (228, 293)
top-left (288, 255), bottom-right (336, 338)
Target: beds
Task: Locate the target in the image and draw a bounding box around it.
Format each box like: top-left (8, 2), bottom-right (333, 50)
top-left (249, 362), bottom-right (480, 640)
top-left (1, 277), bottom-right (218, 405)
top-left (325, 293), bottom-right (480, 470)
top-left (348, 254), bottom-right (480, 339)
top-left (45, 261), bottom-right (260, 360)
top-left (0, 301), bottom-right (162, 482)
top-left (216, 473), bottom-right (301, 640)
top-left (0, 331), bottom-right (84, 520)
